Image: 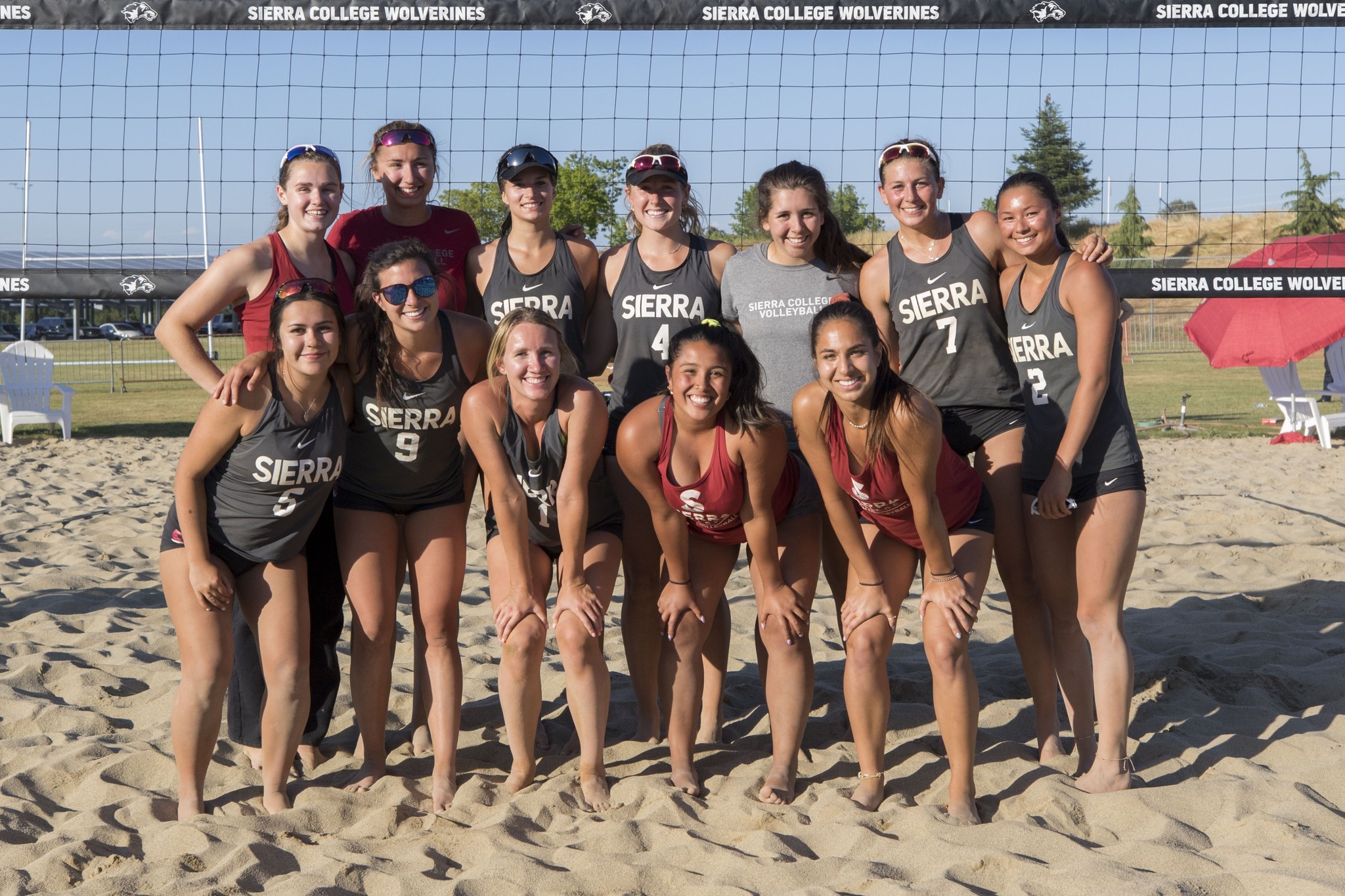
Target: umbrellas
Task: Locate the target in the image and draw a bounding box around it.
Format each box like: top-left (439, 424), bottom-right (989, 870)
top-left (1182, 233), bottom-right (1345, 371)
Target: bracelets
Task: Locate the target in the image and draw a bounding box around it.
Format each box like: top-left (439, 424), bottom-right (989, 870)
top-left (931, 567), bottom-right (955, 576)
top-left (931, 574), bottom-right (959, 582)
top-left (670, 579), bottom-right (691, 584)
top-left (860, 580), bottom-right (883, 586)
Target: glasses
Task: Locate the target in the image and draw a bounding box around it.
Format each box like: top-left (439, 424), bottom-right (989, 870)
top-left (374, 129), bottom-right (435, 153)
top-left (498, 146), bottom-right (557, 186)
top-left (1031, 498), bottom-right (1077, 516)
top-left (279, 144), bottom-right (340, 177)
top-left (625, 154), bottom-right (688, 183)
top-left (374, 275), bottom-right (439, 306)
top-left (878, 142), bottom-right (940, 169)
top-left (273, 278), bottom-right (339, 299)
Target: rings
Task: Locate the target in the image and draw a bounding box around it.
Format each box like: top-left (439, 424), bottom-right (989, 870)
top-left (888, 616), bottom-right (896, 619)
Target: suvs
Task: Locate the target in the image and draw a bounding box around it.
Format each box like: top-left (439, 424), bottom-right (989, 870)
top-left (198, 313), bottom-right (241, 334)
top-left (0, 317), bottom-right (104, 340)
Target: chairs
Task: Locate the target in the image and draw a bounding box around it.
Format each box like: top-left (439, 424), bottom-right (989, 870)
top-left (1258, 337), bottom-right (1345, 449)
top-left (0, 339), bottom-right (74, 445)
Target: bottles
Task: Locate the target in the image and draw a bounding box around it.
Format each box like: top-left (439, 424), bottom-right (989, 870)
top-left (1254, 403), bottom-right (1268, 408)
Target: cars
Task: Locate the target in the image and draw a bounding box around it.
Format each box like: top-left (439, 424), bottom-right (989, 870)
top-left (99, 322), bottom-right (156, 341)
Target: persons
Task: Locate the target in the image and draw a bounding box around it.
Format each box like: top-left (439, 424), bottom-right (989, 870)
top-left (151, 121), bottom-right (1147, 830)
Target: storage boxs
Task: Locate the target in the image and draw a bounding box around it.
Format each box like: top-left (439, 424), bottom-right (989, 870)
top-left (1262, 417), bottom-right (1282, 424)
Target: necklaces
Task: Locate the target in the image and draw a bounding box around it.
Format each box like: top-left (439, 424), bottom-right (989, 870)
top-left (840, 407), bottom-right (868, 429)
top-left (638, 232), bottom-right (685, 255)
top-left (899, 218), bottom-right (943, 263)
top-left (282, 367), bottom-right (321, 422)
top-left (911, 213), bottom-right (942, 252)
